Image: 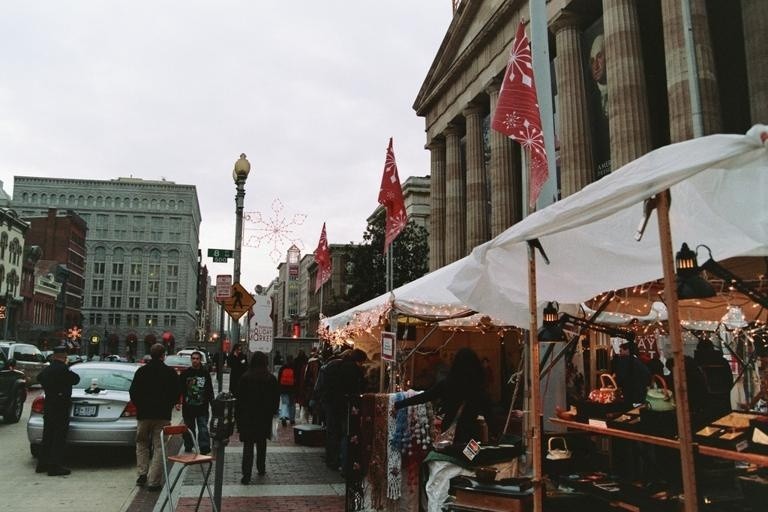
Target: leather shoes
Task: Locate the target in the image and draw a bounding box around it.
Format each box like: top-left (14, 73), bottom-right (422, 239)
top-left (35, 463), bottom-right (48, 473)
top-left (46, 465), bottom-right (72, 476)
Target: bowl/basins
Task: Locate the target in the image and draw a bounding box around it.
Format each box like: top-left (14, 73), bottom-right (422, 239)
top-left (476, 467), bottom-right (497, 483)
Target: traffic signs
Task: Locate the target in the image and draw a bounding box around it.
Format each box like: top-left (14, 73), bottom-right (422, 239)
top-left (208, 248), bottom-right (234, 258)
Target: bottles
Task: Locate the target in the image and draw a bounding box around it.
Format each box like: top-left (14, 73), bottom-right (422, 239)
top-left (91, 378), bottom-right (98, 390)
top-left (475, 418), bottom-right (490, 445)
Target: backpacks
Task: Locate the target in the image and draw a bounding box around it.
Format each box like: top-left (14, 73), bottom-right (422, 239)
top-left (279, 364), bottom-right (296, 386)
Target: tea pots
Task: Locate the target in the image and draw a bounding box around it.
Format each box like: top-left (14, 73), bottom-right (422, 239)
top-left (545, 436), bottom-right (571, 460)
top-left (645, 374), bottom-right (676, 411)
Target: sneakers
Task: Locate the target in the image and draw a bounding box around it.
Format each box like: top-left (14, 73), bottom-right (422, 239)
top-left (240, 472), bottom-right (253, 484)
top-left (143, 484), bottom-right (162, 492)
top-left (184, 446), bottom-right (194, 452)
top-left (290, 420), bottom-right (297, 425)
top-left (281, 417), bottom-right (289, 428)
top-left (257, 469), bottom-right (267, 476)
top-left (199, 448), bottom-right (211, 455)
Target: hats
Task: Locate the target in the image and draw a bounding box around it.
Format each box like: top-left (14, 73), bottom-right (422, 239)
top-left (349, 348), bottom-right (372, 362)
top-left (52, 344), bottom-right (68, 353)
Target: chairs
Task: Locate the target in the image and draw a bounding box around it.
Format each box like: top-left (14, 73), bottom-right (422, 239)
top-left (160, 426), bottom-right (218, 512)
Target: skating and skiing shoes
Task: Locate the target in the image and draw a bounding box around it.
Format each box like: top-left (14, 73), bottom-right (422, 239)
top-left (135, 474), bottom-right (147, 486)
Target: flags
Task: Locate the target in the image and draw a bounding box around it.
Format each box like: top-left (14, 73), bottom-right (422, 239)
top-left (312, 220), bottom-right (335, 297)
top-left (491, 17), bottom-right (553, 207)
top-left (375, 135), bottom-right (410, 256)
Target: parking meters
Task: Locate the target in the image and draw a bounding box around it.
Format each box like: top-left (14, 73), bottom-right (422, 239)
top-left (211, 391), bottom-right (237, 512)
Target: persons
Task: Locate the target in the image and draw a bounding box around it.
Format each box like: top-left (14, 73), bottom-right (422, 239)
top-left (128, 342), bottom-right (182, 493)
top-left (390, 346), bottom-right (490, 446)
top-left (272, 348), bottom-right (370, 473)
top-left (227, 341), bottom-right (249, 396)
top-left (176, 351), bottom-right (216, 456)
top-left (586, 33), bottom-right (610, 151)
top-left (234, 349), bottom-right (280, 484)
top-left (34, 344), bottom-right (81, 476)
top-left (608, 340), bottom-right (649, 404)
top-left (685, 337), bottom-right (732, 420)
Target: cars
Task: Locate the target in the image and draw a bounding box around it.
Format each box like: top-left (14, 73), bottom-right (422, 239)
top-left (0, 338), bottom-right (211, 456)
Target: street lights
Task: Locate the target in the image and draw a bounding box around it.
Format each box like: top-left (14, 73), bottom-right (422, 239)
top-left (224, 150), bottom-right (253, 359)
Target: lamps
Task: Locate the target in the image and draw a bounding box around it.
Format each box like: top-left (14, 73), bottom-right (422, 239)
top-left (676, 242), bottom-right (768, 310)
top-left (538, 301), bottom-right (635, 344)
